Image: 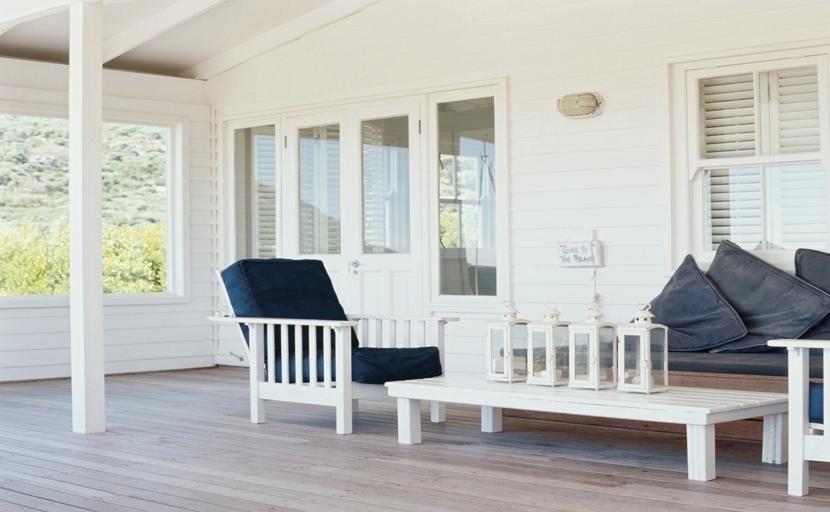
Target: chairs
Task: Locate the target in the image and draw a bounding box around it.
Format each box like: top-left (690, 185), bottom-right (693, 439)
top-left (767, 339), bottom-right (830, 497)
top-left (208, 256), bottom-right (460, 435)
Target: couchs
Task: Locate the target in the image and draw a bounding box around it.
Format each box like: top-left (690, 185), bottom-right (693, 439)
top-left (495, 249), bottom-right (830, 444)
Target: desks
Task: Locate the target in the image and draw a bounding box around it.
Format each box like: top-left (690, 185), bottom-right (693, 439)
top-left (385, 372), bottom-right (789, 481)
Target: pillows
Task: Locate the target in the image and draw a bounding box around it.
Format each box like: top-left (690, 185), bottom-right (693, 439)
top-left (624, 239), bottom-right (830, 358)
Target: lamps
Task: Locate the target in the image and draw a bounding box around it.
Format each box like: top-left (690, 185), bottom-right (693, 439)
top-left (555, 91), bottom-right (606, 120)
